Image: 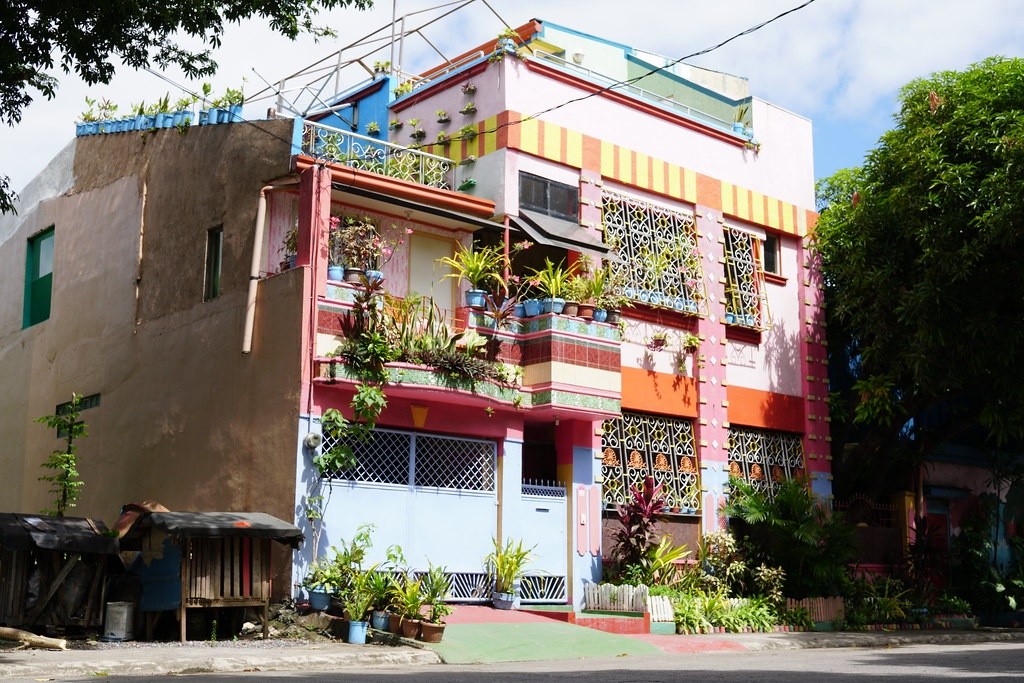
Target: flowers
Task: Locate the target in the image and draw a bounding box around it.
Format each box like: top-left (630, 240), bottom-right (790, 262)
top-left (487, 240), bottom-right (540, 304)
top-left (329, 216), bottom-right (414, 269)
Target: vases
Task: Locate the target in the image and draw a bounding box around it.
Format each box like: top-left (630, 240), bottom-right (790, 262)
top-left (345, 268), bottom-right (364, 286)
top-left (486, 294), bottom-right (509, 313)
top-left (366, 271), bottom-right (383, 286)
top-left (327, 267), bottom-right (344, 282)
top-left (511, 304), bottom-right (526, 318)
top-left (523, 299), bottom-right (543, 317)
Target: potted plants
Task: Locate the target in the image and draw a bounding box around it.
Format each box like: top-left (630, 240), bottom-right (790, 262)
top-left (303, 384), bottom-right (454, 645)
top-left (646, 332), bottom-right (668, 352)
top-left (863, 575), bottom-right (954, 631)
top-left (498, 28), bottom-right (519, 50)
top-left (208, 99), bottom-right (223, 125)
top-left (603, 243), bottom-right (755, 326)
top-left (577, 254), bottom-right (596, 319)
top-left (217, 97), bottom-right (229, 124)
top-left (434, 240), bottom-right (508, 309)
top-left (683, 333), bottom-right (701, 354)
top-left (373, 61), bottom-right (390, 80)
top-left (227, 77), bottom-right (247, 122)
top-left (483, 536), bottom-right (539, 609)
top-left (732, 104), bottom-right (754, 138)
top-left (675, 586), bottom-right (812, 634)
top-left (198, 83), bottom-right (212, 126)
top-left (277, 228), bottom-right (299, 271)
top-left (521, 256), bottom-right (582, 314)
top-left (592, 269), bottom-right (608, 323)
top-left (77, 93), bottom-right (198, 136)
top-left (561, 277), bottom-right (586, 317)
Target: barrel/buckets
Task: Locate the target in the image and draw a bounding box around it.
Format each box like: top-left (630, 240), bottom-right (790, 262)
top-left (100, 602), bottom-right (135, 642)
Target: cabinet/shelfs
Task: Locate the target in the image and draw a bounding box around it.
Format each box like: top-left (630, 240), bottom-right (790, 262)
top-left (367, 85), bottom-right (476, 191)
